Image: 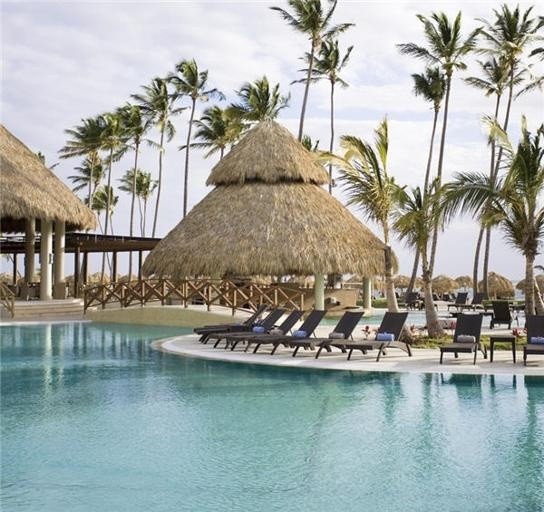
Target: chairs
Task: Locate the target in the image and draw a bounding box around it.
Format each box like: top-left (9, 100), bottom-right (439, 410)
top-left (290, 311), bottom-right (366, 359)
top-left (439, 313), bottom-right (487, 366)
top-left (488, 300), bottom-right (520, 330)
top-left (447, 292), bottom-right (468, 312)
top-left (523, 315), bottom-right (544, 365)
top-left (405, 292), bottom-right (457, 311)
top-left (462, 293), bottom-right (486, 312)
top-left (251, 309), bottom-right (329, 355)
top-left (195, 305), bottom-right (307, 356)
top-left (344, 312), bottom-right (413, 363)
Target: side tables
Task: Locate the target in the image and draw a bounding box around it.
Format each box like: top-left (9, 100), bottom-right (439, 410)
top-left (489, 330), bottom-right (518, 364)
